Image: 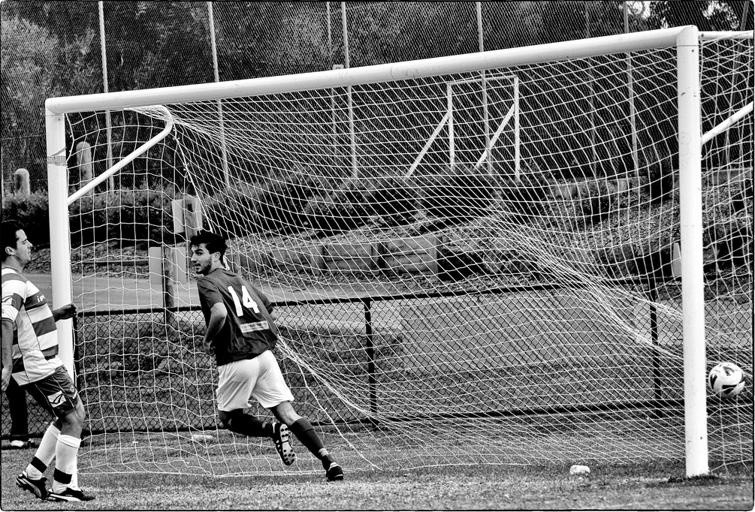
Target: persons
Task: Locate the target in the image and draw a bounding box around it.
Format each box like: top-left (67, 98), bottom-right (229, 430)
top-left (189, 230), bottom-right (344, 482)
top-left (1, 220), bottom-right (96, 501)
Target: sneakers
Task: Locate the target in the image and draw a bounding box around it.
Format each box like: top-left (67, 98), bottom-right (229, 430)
top-left (270, 421), bottom-right (297, 466)
top-left (15, 470), bottom-right (49, 501)
top-left (10, 438), bottom-right (35, 448)
top-left (326, 461), bottom-right (344, 481)
top-left (44, 486), bottom-right (96, 503)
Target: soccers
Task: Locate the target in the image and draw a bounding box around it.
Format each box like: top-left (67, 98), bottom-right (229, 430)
top-left (707, 361), bottom-right (745, 397)
